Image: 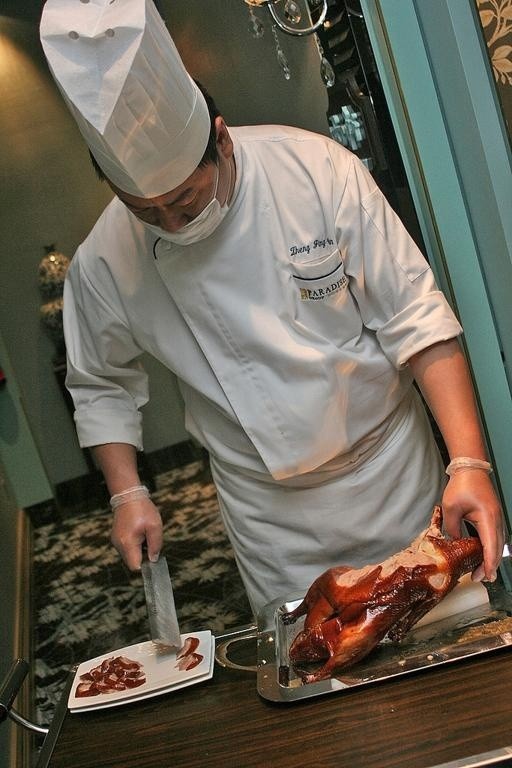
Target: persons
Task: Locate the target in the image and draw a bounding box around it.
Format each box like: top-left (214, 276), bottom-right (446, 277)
top-left (59, 73), bottom-right (511, 585)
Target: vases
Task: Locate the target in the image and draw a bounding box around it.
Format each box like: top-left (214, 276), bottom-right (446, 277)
top-left (36, 236), bottom-right (73, 352)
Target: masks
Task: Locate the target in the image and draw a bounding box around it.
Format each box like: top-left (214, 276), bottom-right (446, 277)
top-left (115, 161), bottom-right (239, 250)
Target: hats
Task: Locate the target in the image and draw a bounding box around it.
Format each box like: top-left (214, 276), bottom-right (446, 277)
top-left (37, 2), bottom-right (215, 203)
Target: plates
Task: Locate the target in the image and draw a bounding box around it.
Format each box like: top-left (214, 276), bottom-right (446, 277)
top-left (66, 629), bottom-right (216, 715)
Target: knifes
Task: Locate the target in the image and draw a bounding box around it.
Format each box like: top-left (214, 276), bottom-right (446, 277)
top-left (139, 554), bottom-right (184, 653)
